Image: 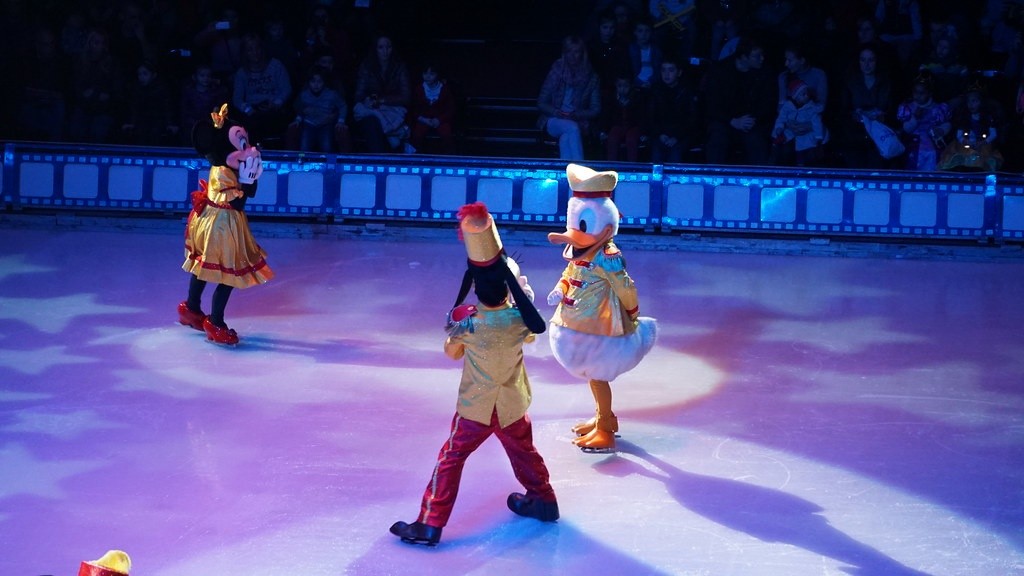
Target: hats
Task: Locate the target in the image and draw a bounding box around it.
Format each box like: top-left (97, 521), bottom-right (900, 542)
top-left (788, 81), bottom-right (809, 99)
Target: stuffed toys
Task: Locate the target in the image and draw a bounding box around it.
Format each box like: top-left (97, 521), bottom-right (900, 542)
top-left (542, 165), bottom-right (656, 451)
top-left (382, 204), bottom-right (560, 546)
top-left (176, 102), bottom-right (274, 347)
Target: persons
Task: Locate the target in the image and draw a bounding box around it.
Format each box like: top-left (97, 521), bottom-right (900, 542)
top-left (2, 0), bottom-right (1024, 180)
